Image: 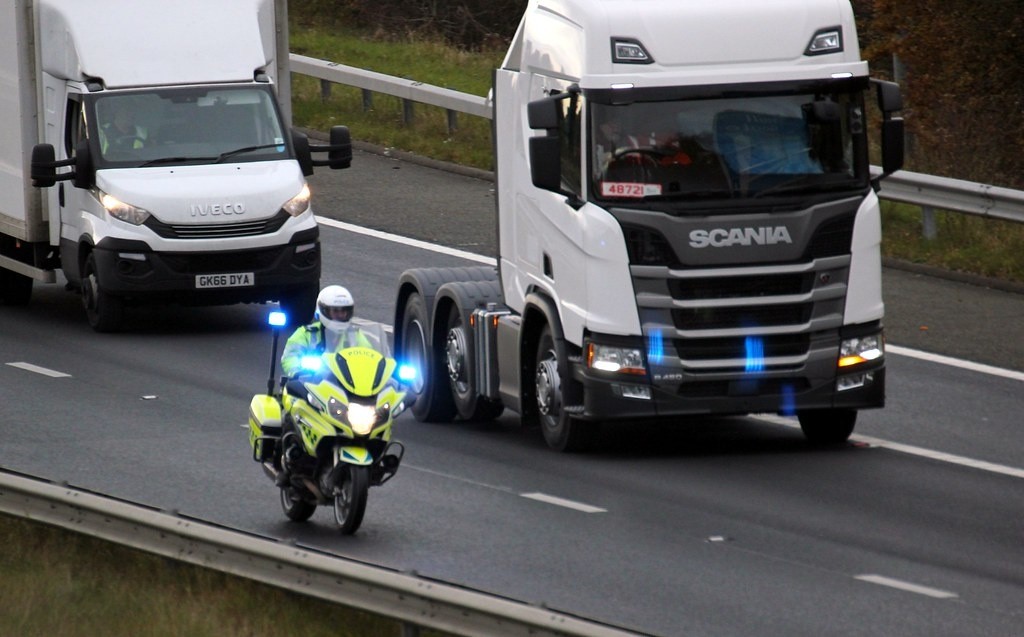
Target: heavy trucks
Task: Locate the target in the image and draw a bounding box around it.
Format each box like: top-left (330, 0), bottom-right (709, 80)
top-left (393, 0), bottom-right (907, 455)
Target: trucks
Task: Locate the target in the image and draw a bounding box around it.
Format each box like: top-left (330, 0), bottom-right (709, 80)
top-left (0, 0), bottom-right (351, 334)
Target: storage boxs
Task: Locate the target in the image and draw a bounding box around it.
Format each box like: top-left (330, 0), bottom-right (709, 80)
top-left (248, 394), bottom-right (282, 458)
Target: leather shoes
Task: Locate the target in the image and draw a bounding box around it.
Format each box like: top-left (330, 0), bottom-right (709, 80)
top-left (275, 473), bottom-right (291, 487)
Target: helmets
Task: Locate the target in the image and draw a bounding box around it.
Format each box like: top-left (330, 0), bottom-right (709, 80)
top-left (315, 285), bottom-right (355, 333)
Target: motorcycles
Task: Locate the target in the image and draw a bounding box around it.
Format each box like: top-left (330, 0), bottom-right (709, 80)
top-left (248, 311), bottom-right (421, 538)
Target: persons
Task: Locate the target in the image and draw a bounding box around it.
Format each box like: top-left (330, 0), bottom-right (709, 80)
top-left (279, 285), bottom-right (375, 492)
top-left (97, 96), bottom-right (149, 158)
top-left (587, 102), bottom-right (666, 191)
